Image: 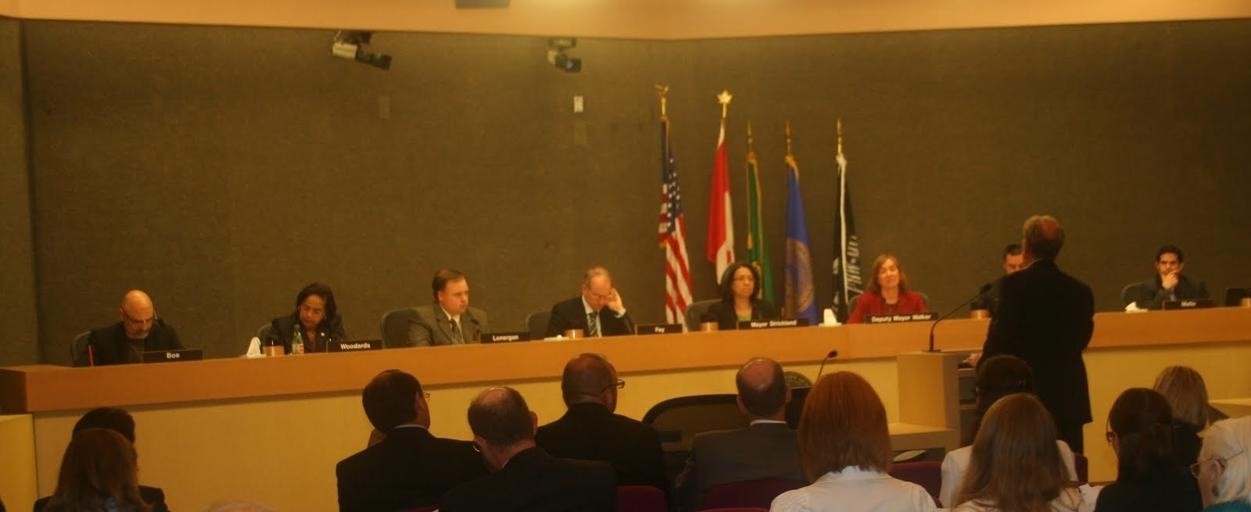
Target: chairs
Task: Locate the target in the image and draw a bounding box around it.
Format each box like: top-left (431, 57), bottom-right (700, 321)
top-left (705, 479), bottom-right (815, 512)
top-left (886, 460), bottom-right (947, 506)
top-left (615, 485), bottom-right (665, 512)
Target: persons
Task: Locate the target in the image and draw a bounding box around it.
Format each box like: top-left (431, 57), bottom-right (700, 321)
top-left (46, 427), bottom-right (150, 511)
top-left (260, 282), bottom-right (348, 356)
top-left (674, 356), bottom-right (799, 500)
top-left (708, 260), bottom-right (777, 331)
top-left (938, 354), bottom-right (1080, 510)
top-left (770, 371), bottom-right (937, 512)
top-left (32, 407), bottom-right (170, 511)
top-left (405, 265), bottom-right (486, 348)
top-left (1153, 364), bottom-right (1229, 431)
top-left (550, 265), bottom-right (636, 340)
top-left (466, 384), bottom-right (615, 512)
top-left (71, 286), bottom-right (185, 367)
top-left (845, 252), bottom-right (929, 324)
top-left (534, 351), bottom-right (669, 490)
top-left (1095, 388), bottom-right (1202, 512)
top-left (951, 392), bottom-right (1086, 512)
top-left (336, 368), bottom-right (481, 512)
top-left (977, 243), bottom-right (1027, 319)
top-left (1194, 414), bottom-right (1251, 512)
top-left (964, 212), bottom-right (1094, 457)
top-left (1135, 244), bottom-right (1213, 311)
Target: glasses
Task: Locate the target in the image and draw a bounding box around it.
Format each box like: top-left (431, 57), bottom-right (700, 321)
top-left (297, 305), bottom-right (322, 316)
top-left (586, 284), bottom-right (612, 301)
top-left (473, 442), bottom-right (480, 452)
top-left (424, 392), bottom-right (430, 398)
top-left (1106, 431), bottom-right (1114, 442)
top-left (1189, 449), bottom-right (1243, 478)
top-left (601, 378), bottom-right (625, 392)
top-left (120, 306), bottom-right (154, 323)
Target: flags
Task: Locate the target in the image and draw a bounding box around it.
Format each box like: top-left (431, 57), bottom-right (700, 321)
top-left (743, 118), bottom-right (774, 305)
top-left (654, 84), bottom-right (697, 333)
top-left (705, 90), bottom-right (736, 287)
top-left (830, 118), bottom-right (864, 323)
top-left (782, 122), bottom-right (820, 326)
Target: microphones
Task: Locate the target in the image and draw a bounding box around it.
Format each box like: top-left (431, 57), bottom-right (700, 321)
top-left (928, 282), bottom-right (994, 353)
top-left (818, 350), bottom-right (838, 378)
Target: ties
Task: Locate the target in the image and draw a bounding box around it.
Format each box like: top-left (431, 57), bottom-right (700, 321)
top-left (449, 319), bottom-right (463, 343)
top-left (589, 311), bottom-right (598, 336)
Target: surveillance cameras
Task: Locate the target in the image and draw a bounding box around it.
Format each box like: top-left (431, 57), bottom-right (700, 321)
top-left (330, 29), bottom-right (392, 70)
top-left (547, 38), bottom-right (583, 74)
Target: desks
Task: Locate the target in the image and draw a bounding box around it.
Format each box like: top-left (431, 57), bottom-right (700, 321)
top-left (3, 307), bottom-right (1251, 512)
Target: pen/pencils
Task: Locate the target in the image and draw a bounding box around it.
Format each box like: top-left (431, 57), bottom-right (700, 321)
top-left (87, 344), bottom-right (95, 367)
